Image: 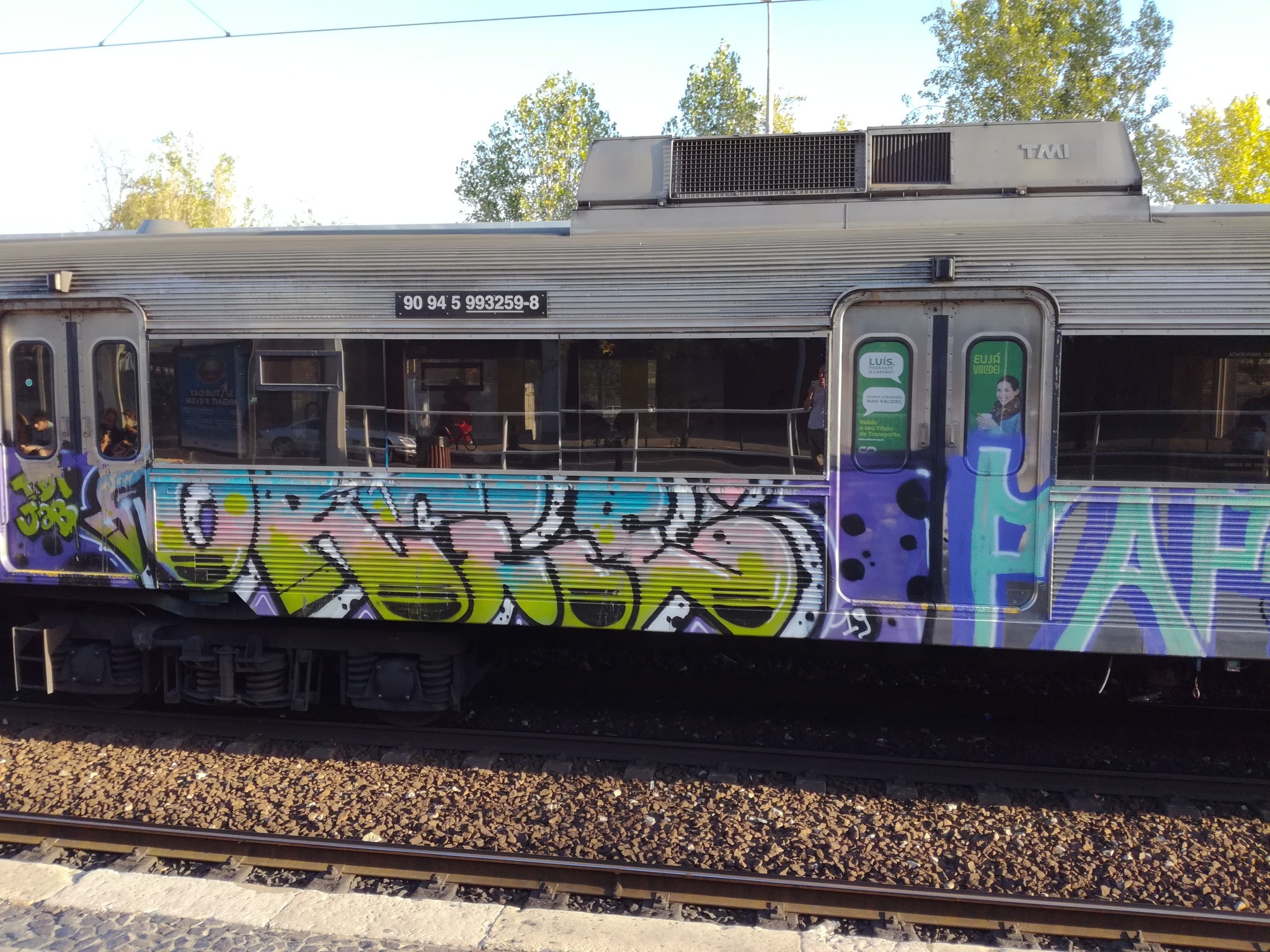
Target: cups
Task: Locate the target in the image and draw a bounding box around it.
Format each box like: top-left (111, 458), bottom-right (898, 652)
top-left (981, 413), bottom-right (992, 430)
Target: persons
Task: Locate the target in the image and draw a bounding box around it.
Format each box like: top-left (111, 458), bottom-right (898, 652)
top-left (803, 363), bottom-right (825, 472)
top-left (974, 375), bottom-right (1021, 438)
top-left (431, 377), bottom-right (470, 444)
top-left (95, 370), bottom-right (137, 460)
top-left (17, 410), bottom-right (54, 457)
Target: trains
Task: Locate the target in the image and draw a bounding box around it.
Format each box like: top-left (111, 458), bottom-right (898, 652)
top-left (0, 119), bottom-right (1270, 706)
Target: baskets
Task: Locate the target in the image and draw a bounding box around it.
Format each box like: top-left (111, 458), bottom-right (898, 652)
top-left (460, 424), bottom-right (472, 432)
